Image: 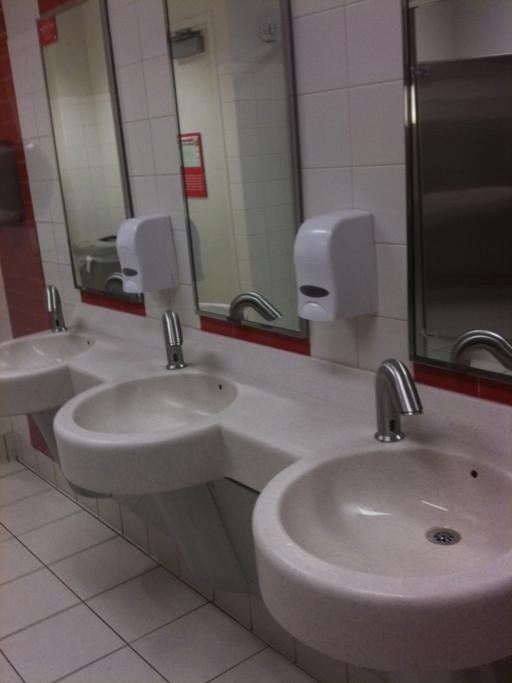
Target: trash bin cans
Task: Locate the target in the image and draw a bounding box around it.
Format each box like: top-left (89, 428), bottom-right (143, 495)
top-left (71, 235), bottom-right (138, 302)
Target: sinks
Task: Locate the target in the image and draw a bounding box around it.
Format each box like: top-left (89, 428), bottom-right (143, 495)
top-left (1, 328), bottom-right (98, 415)
top-left (51, 373), bottom-right (240, 497)
top-left (249, 444), bottom-right (512, 674)
top-left (198, 301), bottom-right (231, 317)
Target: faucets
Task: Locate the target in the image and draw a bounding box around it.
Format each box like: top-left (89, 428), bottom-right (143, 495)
top-left (449, 329), bottom-right (512, 373)
top-left (372, 358), bottom-right (423, 443)
top-left (102, 274), bottom-right (142, 303)
top-left (44, 285), bottom-right (68, 333)
top-left (161, 309), bottom-right (189, 369)
top-left (226, 292), bottom-right (283, 322)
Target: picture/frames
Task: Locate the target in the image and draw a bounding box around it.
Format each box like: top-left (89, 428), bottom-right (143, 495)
top-left (161, 1), bottom-right (313, 342)
top-left (37, 0), bottom-right (148, 311)
top-left (396, 0), bottom-right (511, 385)
